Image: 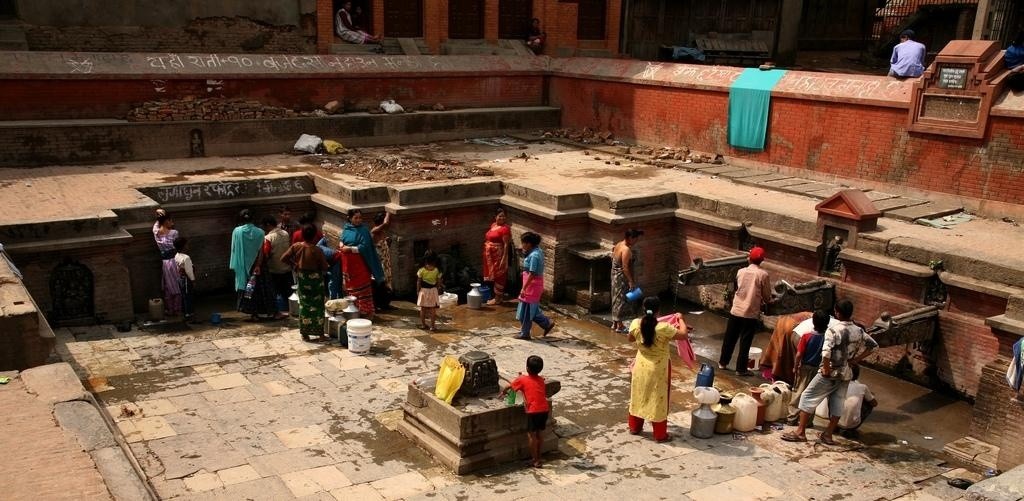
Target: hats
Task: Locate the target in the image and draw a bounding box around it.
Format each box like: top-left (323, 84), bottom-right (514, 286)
top-left (749, 245), bottom-right (766, 261)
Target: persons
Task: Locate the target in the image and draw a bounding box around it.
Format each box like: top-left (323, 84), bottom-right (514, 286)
top-left (718, 247), bottom-right (774, 376)
top-left (279, 205), bottom-right (295, 232)
top-left (264, 215), bottom-right (294, 300)
top-left (315, 238), bottom-right (344, 299)
top-left (417, 250), bottom-right (443, 331)
top-left (335, 1), bottom-right (383, 44)
top-left (828, 364), bottom-right (877, 436)
top-left (229, 214), bottom-right (288, 321)
top-left (524, 18), bottom-right (546, 54)
top-left (760, 309), bottom-right (816, 382)
top-left (786, 309), bottom-right (833, 426)
top-left (627, 296), bottom-right (690, 442)
top-left (292, 213), bottom-right (325, 283)
top-left (887, 30), bottom-right (926, 77)
top-left (151, 207), bottom-right (179, 316)
top-left (514, 232), bottom-right (556, 339)
top-left (527, 32), bottom-right (548, 46)
top-left (611, 228), bottom-right (644, 334)
top-left (369, 210), bottom-right (399, 311)
top-left (497, 354), bottom-right (549, 468)
top-left (280, 224), bottom-right (333, 341)
top-left (172, 237), bottom-right (198, 324)
top-left (483, 207), bottom-right (512, 306)
top-left (338, 209), bottom-right (384, 319)
top-left (791, 314), bottom-right (866, 393)
top-left (781, 298), bottom-right (879, 446)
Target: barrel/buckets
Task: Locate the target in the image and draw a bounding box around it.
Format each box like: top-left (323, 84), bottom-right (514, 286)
top-left (479, 286), bottom-right (491, 300)
top-left (346, 318), bottom-right (372, 355)
top-left (760, 383), bottom-right (782, 421)
top-left (746, 346), bottom-right (763, 371)
top-left (730, 392), bottom-right (758, 432)
top-left (773, 380), bottom-right (792, 418)
top-left (695, 363), bottom-right (714, 387)
top-left (438, 293), bottom-right (458, 308)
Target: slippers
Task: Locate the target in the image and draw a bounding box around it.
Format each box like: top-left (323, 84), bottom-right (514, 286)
top-left (781, 429), bottom-right (808, 443)
top-left (543, 318), bottom-right (556, 337)
top-left (814, 429), bottom-right (835, 446)
top-left (512, 334), bottom-right (532, 340)
